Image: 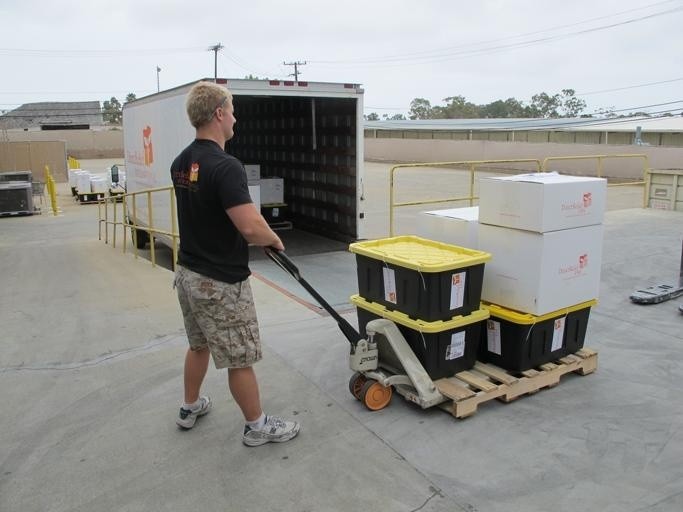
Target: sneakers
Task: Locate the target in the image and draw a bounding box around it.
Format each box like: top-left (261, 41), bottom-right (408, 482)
top-left (242, 414), bottom-right (301, 448)
top-left (176, 394), bottom-right (213, 429)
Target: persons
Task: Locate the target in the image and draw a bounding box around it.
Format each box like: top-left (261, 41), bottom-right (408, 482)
top-left (172, 80), bottom-right (300, 446)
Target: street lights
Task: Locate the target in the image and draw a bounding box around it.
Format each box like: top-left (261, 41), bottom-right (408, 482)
top-left (156, 65), bottom-right (162, 92)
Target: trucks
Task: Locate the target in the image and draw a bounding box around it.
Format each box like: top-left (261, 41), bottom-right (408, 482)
top-left (107, 70), bottom-right (374, 276)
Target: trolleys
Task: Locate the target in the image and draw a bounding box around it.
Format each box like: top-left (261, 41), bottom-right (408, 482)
top-left (259, 235), bottom-right (595, 417)
